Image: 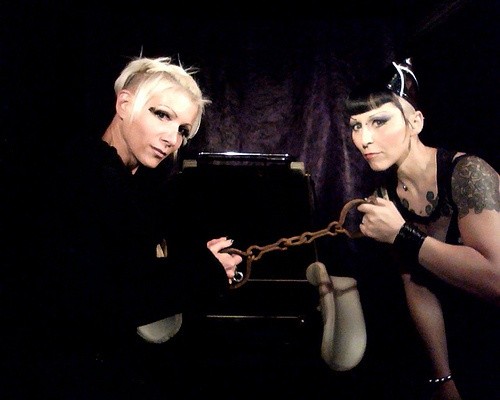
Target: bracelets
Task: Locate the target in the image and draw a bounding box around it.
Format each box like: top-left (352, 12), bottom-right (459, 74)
top-left (426, 375), bottom-right (453, 384)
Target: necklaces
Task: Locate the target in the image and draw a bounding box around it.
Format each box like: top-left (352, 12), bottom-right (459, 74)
top-left (397, 176), bottom-right (408, 191)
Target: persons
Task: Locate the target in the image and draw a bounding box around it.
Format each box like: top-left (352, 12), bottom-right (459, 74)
top-left (11, 47), bottom-right (242, 400)
top-left (341, 58), bottom-right (500, 400)
